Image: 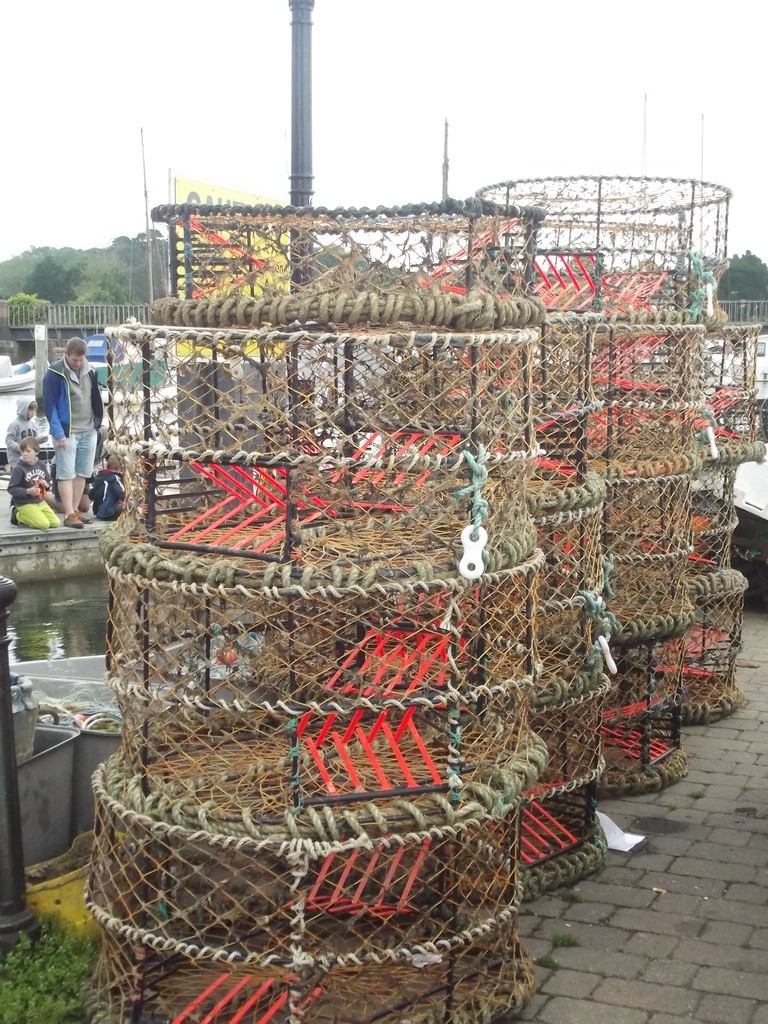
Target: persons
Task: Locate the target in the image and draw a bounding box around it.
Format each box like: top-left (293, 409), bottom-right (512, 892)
top-left (45, 427), bottom-right (111, 514)
top-left (42, 336), bottom-right (102, 528)
top-left (5, 397), bottom-right (48, 470)
top-left (92, 455), bottom-right (142, 520)
top-left (7, 437), bottom-right (61, 528)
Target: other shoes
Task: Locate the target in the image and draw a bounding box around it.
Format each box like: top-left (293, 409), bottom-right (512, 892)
top-left (10, 505), bottom-right (19, 527)
top-left (64, 511), bottom-right (93, 528)
